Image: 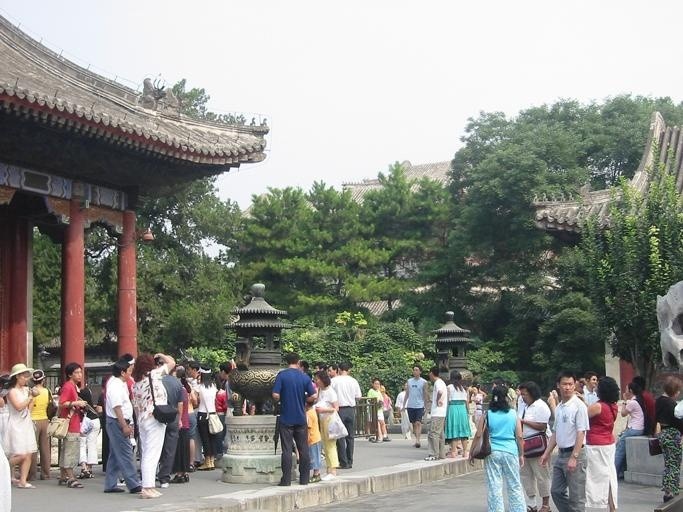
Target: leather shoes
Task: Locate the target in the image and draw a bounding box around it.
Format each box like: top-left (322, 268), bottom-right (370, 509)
top-left (169, 475), bottom-right (184, 483)
top-left (103, 487), bottom-right (124, 493)
top-left (184, 474), bottom-right (188, 481)
top-left (129, 485), bottom-right (141, 492)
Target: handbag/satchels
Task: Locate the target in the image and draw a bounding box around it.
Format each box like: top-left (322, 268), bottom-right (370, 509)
top-left (46, 387), bottom-right (57, 421)
top-left (46, 416), bottom-right (70, 439)
top-left (20, 407), bottom-right (30, 420)
top-left (152, 404), bottom-right (177, 424)
top-left (205, 412), bottom-right (223, 434)
top-left (648, 437), bottom-right (662, 456)
top-left (522, 432), bottom-right (547, 457)
top-left (468, 410), bottom-right (490, 460)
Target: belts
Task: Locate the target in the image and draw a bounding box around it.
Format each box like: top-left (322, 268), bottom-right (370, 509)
top-left (558, 444), bottom-right (583, 452)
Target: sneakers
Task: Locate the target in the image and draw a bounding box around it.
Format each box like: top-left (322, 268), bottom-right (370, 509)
top-left (151, 488), bottom-right (161, 495)
top-left (16, 483), bottom-right (32, 488)
top-left (424, 455), bottom-right (439, 461)
top-left (197, 464), bottom-right (211, 471)
top-left (140, 489), bottom-right (158, 498)
top-left (160, 483), bottom-right (169, 489)
top-left (211, 464), bottom-right (214, 470)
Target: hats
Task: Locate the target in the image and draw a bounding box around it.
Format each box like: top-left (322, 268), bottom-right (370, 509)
top-left (32, 369), bottom-right (45, 381)
top-left (128, 358), bottom-right (134, 365)
top-left (9, 363), bottom-right (34, 380)
top-left (197, 367), bottom-right (211, 374)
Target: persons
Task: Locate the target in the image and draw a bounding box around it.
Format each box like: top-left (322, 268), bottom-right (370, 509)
top-left (468, 371), bottom-right (683, 511)
top-left (271, 353), bottom-right (361, 486)
top-left (366, 364), bottom-right (471, 461)
top-left (1, 353), bottom-right (256, 511)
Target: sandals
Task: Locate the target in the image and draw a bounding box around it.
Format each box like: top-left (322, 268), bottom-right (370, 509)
top-left (309, 476), bottom-right (319, 483)
top-left (537, 505), bottom-right (550, 512)
top-left (527, 505), bottom-right (537, 512)
top-left (189, 465), bottom-right (195, 472)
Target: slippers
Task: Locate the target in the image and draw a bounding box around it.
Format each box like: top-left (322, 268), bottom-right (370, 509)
top-left (66, 480), bottom-right (82, 488)
top-left (58, 479), bottom-right (67, 485)
